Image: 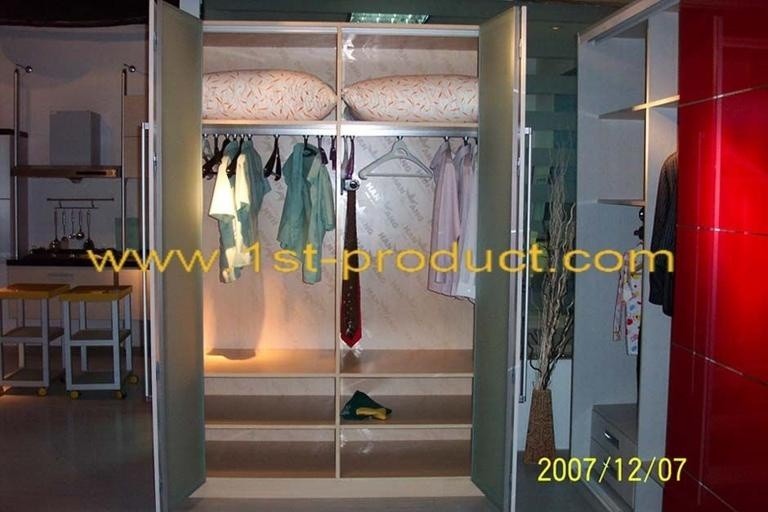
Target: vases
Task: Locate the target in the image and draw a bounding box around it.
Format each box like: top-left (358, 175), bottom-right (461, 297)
top-left (524, 383), bottom-right (559, 475)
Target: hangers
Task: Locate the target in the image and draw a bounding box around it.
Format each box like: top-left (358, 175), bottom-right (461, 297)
top-left (199, 132), bottom-right (480, 182)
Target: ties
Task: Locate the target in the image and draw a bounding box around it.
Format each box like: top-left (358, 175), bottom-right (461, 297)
top-left (341, 188), bottom-right (363, 349)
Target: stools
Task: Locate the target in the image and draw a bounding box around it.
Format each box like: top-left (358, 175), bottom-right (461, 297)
top-left (60, 283), bottom-right (134, 400)
top-left (0, 280), bottom-right (69, 396)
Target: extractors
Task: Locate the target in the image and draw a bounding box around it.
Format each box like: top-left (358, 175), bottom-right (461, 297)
top-left (9, 111), bottom-right (123, 183)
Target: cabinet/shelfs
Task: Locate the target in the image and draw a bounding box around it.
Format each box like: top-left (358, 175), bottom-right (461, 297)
top-left (141, 0), bottom-right (532, 512)
top-left (576, 2), bottom-right (768, 511)
top-left (120, 95), bottom-right (149, 179)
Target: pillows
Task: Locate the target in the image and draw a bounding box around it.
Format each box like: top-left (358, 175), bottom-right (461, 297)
top-left (339, 72), bottom-right (481, 125)
top-left (200, 68), bottom-right (336, 124)
